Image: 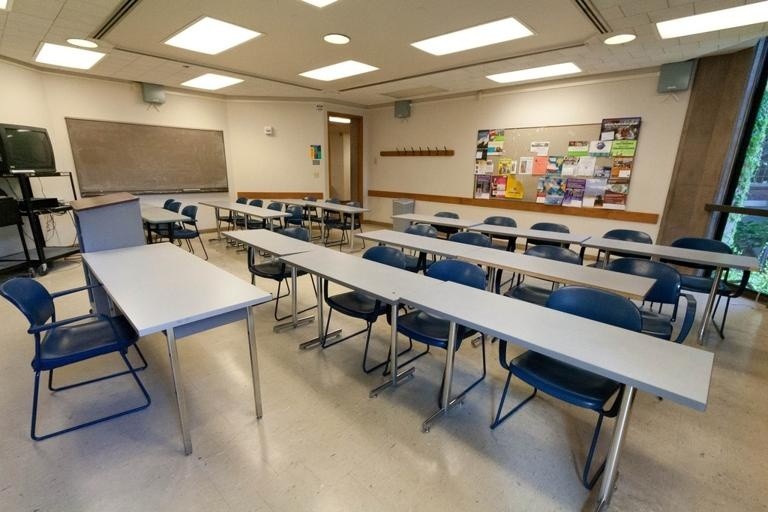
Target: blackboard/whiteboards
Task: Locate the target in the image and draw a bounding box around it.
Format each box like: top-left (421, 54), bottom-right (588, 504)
top-left (64, 117), bottom-right (229, 199)
top-left (473, 117), bottom-right (642, 211)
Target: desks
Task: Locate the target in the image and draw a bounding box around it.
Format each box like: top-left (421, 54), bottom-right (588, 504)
top-left (79, 242), bottom-right (273, 456)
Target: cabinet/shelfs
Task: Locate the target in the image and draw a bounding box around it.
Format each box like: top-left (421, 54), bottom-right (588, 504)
top-left (0, 173), bottom-right (80, 279)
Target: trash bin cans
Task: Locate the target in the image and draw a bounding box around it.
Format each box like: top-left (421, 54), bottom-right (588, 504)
top-left (392, 198), bottom-right (414, 233)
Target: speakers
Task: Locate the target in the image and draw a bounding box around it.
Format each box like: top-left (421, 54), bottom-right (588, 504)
top-left (657, 59), bottom-right (695, 93)
top-left (142, 84), bottom-right (166, 104)
top-left (393, 100), bottom-right (411, 118)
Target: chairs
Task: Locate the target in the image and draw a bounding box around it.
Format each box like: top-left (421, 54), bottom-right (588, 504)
top-left (0, 278), bottom-right (151, 442)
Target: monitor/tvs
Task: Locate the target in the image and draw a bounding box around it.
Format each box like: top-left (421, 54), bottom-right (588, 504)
top-left (0, 123), bottom-right (57, 174)
